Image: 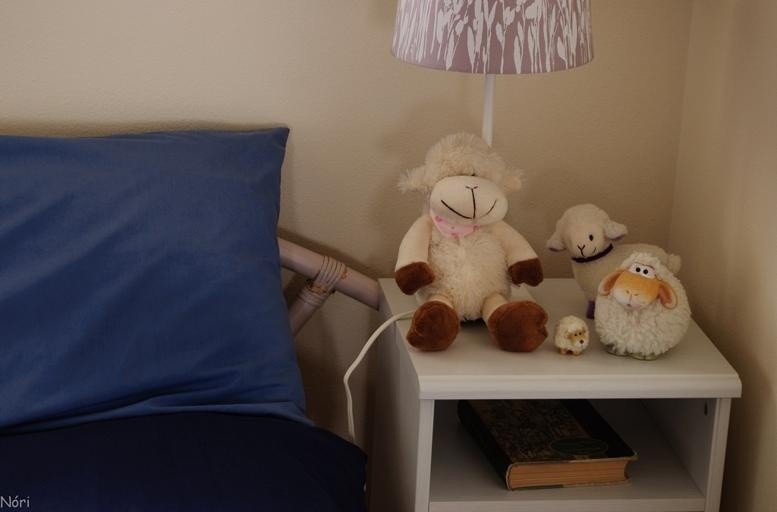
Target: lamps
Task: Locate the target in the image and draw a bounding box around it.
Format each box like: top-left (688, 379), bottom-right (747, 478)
top-left (390, 1), bottom-right (596, 327)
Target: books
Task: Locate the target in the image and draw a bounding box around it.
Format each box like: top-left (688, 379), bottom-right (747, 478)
top-left (453, 398), bottom-right (639, 494)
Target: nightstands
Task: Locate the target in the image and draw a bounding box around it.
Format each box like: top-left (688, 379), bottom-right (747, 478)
top-left (368, 274), bottom-right (745, 512)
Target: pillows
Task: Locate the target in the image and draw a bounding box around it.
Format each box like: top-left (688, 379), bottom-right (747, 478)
top-left (0, 127), bottom-right (315, 427)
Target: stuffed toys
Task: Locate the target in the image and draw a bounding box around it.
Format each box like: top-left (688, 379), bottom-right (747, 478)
top-left (553, 312), bottom-right (591, 356)
top-left (593, 249), bottom-right (692, 362)
top-left (390, 129), bottom-right (548, 355)
top-left (544, 199), bottom-right (682, 321)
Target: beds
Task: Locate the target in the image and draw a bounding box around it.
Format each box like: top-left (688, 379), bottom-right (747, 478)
top-left (0, 236), bottom-right (382, 511)
